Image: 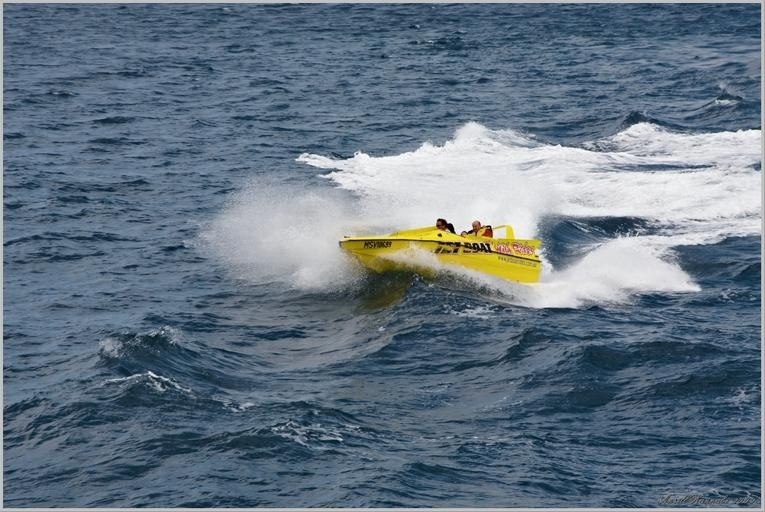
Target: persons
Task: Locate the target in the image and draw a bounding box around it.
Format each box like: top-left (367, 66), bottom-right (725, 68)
top-left (436, 219), bottom-right (451, 233)
top-left (461, 221), bottom-right (492, 237)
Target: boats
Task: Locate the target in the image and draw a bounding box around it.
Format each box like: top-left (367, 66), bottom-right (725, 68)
top-left (338, 215), bottom-right (544, 289)
